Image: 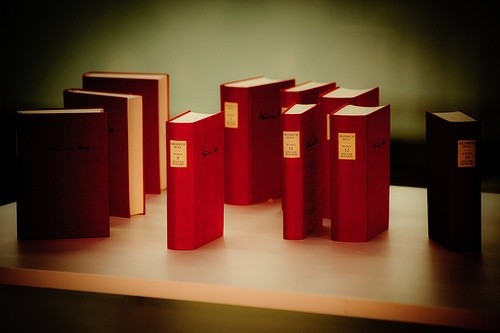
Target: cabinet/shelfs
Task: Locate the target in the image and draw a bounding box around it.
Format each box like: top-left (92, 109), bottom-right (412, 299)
top-left (0, 185), bottom-right (499, 333)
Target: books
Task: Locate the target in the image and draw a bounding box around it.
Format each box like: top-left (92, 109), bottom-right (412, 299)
top-left (60, 86), bottom-right (150, 219)
top-left (278, 99), bottom-right (321, 242)
top-left (327, 101), bottom-right (392, 244)
top-left (423, 107), bottom-right (487, 257)
top-left (278, 79), bottom-right (336, 215)
top-left (317, 84), bottom-right (383, 242)
top-left (217, 72), bottom-right (298, 208)
top-left (79, 68), bottom-right (173, 196)
top-left (165, 107), bottom-right (227, 252)
top-left (14, 105), bottom-right (114, 245)
